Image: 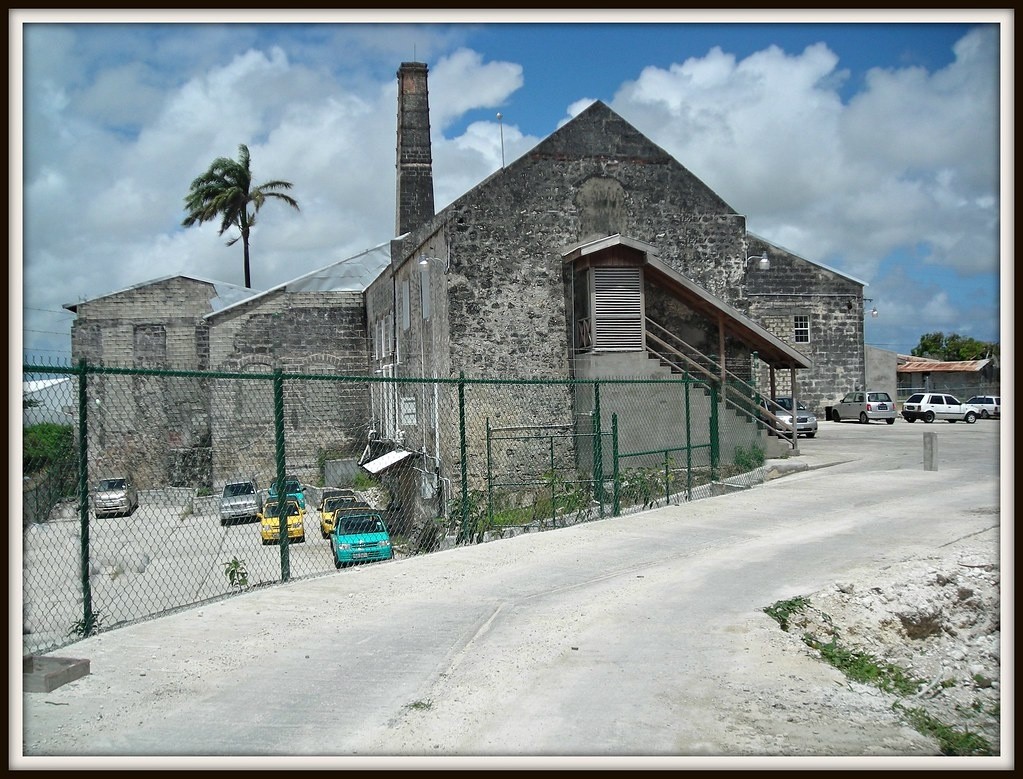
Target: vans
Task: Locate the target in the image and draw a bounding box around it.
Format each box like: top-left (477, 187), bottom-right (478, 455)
top-left (94, 477), bottom-right (139, 516)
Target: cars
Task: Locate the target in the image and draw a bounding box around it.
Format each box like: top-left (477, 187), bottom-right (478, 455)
top-left (269, 475), bottom-right (300, 494)
top-left (316, 490), bottom-right (384, 542)
top-left (256, 501), bottom-right (306, 545)
top-left (964, 395), bottom-right (1000, 420)
top-left (332, 514), bottom-right (397, 568)
top-left (218, 481), bottom-right (264, 525)
top-left (900, 393), bottom-right (982, 424)
top-left (762, 397), bottom-right (819, 437)
top-left (268, 482), bottom-right (308, 514)
top-left (831, 391), bottom-right (897, 424)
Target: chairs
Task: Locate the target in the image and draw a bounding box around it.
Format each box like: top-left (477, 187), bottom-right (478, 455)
top-left (344, 521), bottom-right (356, 533)
top-left (871, 395), bottom-right (876, 401)
top-left (363, 520), bottom-right (379, 533)
top-left (786, 400), bottom-right (791, 409)
top-left (858, 396), bottom-right (864, 402)
top-left (271, 505), bottom-right (278, 516)
top-left (286, 505), bottom-right (297, 516)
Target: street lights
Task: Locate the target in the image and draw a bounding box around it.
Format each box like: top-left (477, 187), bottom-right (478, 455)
top-left (497, 112), bottom-right (508, 170)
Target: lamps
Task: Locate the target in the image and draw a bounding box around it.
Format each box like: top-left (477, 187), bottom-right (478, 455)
top-left (747, 251), bottom-right (770, 270)
top-left (418, 257), bottom-right (446, 271)
top-left (864, 306), bottom-right (878, 318)
